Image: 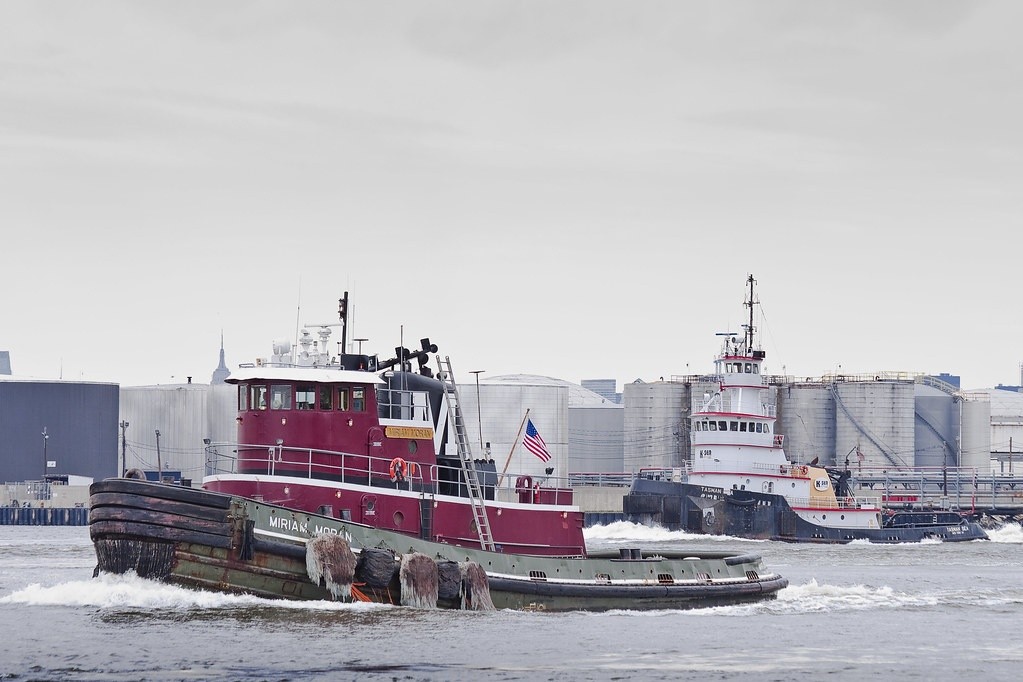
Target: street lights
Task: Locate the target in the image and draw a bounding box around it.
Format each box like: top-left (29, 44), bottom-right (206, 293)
top-left (120, 421), bottom-right (129, 477)
top-left (155, 429), bottom-right (163, 483)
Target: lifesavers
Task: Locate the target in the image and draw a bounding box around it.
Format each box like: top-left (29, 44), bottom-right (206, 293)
top-left (775, 434), bottom-right (783, 445)
top-left (389, 456), bottom-right (407, 481)
top-left (515, 476), bottom-right (532, 494)
top-left (801, 465), bottom-right (809, 475)
top-left (845, 497), bottom-right (854, 506)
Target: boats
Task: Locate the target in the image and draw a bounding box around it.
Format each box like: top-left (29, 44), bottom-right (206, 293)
top-left (623, 272), bottom-right (990, 544)
top-left (88, 288), bottom-right (788, 612)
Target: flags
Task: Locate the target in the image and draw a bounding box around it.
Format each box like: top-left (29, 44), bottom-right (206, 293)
top-left (522, 418), bottom-right (552, 462)
